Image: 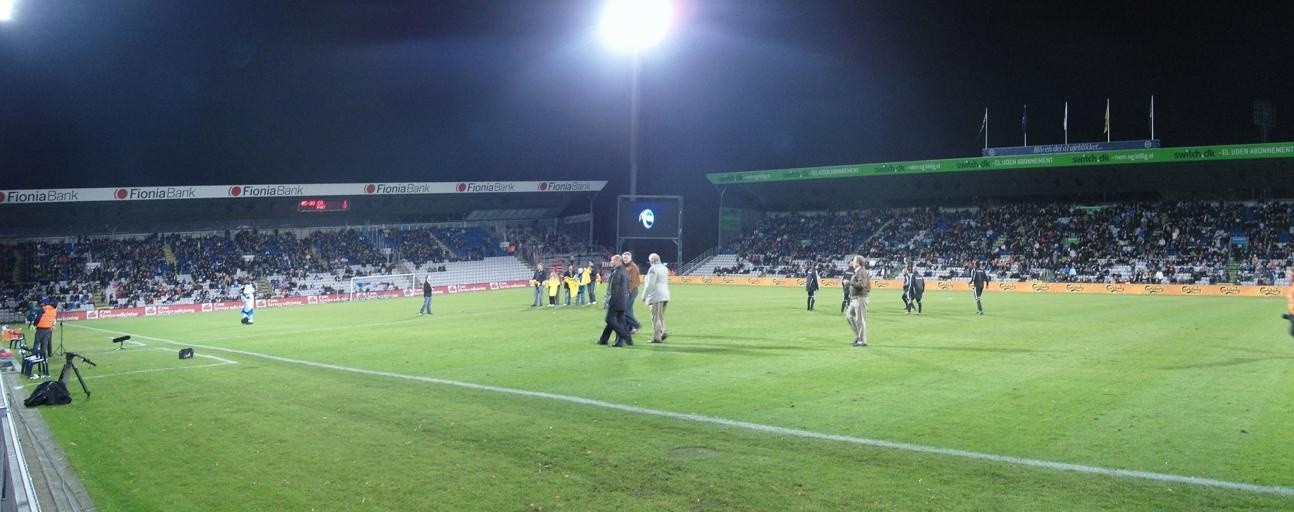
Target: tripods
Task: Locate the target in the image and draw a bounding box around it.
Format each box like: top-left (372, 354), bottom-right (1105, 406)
top-left (53, 321), bottom-right (65, 356)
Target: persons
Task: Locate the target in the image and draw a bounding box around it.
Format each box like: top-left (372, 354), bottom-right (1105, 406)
top-left (34, 300), bottom-right (58, 356)
top-left (846, 254), bottom-right (869, 349)
top-left (47, 319), bottom-right (56, 356)
top-left (238, 280), bottom-right (255, 323)
top-left (714, 203), bottom-right (1293, 285)
top-left (901, 267), bottom-right (925, 315)
top-left (622, 252), bottom-right (641, 333)
top-left (967, 260), bottom-right (988, 315)
top-left (641, 252), bottom-right (670, 343)
top-left (806, 263), bottom-right (820, 311)
top-left (597, 261), bottom-right (625, 347)
top-left (0, 214), bottom-right (497, 313)
top-left (841, 262), bottom-right (855, 313)
top-left (419, 275), bottom-right (432, 315)
top-left (506, 217), bottom-right (588, 261)
top-left (605, 255), bottom-right (633, 346)
top-left (531, 260), bottom-right (596, 306)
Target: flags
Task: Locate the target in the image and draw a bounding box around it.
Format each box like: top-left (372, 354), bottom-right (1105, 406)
top-left (1103, 101), bottom-right (1109, 134)
top-left (1014, 107), bottom-right (1028, 130)
top-left (1146, 100), bottom-right (1153, 129)
top-left (975, 110), bottom-right (987, 136)
top-left (1059, 103), bottom-right (1068, 131)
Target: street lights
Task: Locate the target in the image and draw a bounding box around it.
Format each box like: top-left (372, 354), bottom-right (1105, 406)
top-left (596, 0), bottom-right (676, 201)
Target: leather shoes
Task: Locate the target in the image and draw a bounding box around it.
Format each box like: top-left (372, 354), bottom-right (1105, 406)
top-left (608, 343), bottom-right (624, 347)
top-left (648, 339), bottom-right (661, 343)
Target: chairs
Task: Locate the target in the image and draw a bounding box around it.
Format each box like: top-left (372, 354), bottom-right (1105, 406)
top-left (6, 329), bottom-right (48, 378)
